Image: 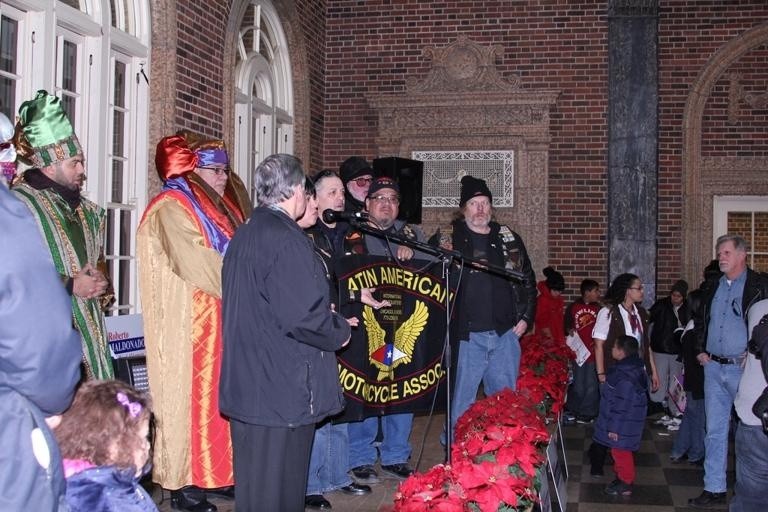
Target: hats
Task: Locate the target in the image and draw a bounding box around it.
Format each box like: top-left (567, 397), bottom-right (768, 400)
top-left (340, 155), bottom-right (373, 183)
top-left (368, 176), bottom-right (403, 196)
top-left (670, 278), bottom-right (689, 299)
top-left (458, 175), bottom-right (493, 209)
top-left (543, 266), bottom-right (566, 292)
top-left (19, 89), bottom-right (82, 169)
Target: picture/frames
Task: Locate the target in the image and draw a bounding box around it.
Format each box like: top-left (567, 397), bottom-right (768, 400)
top-left (408, 144), bottom-right (519, 213)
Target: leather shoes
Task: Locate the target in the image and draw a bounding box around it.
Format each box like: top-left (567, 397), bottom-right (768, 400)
top-left (670, 456), bottom-right (704, 466)
top-left (688, 490), bottom-right (728, 508)
top-left (351, 463), bottom-right (378, 484)
top-left (204, 485), bottom-right (237, 501)
top-left (590, 463), bottom-right (604, 477)
top-left (344, 482), bottom-right (372, 496)
top-left (381, 463), bottom-right (416, 480)
top-left (170, 497), bottom-right (218, 512)
top-left (306, 492), bottom-right (333, 512)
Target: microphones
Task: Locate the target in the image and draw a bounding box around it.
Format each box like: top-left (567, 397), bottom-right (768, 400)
top-left (321, 207), bottom-right (373, 226)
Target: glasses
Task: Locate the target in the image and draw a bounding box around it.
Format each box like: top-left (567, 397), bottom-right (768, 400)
top-left (369, 195), bottom-right (399, 204)
top-left (732, 298), bottom-right (743, 318)
top-left (351, 178), bottom-right (375, 187)
top-left (197, 166), bottom-right (229, 175)
top-left (629, 285), bottom-right (643, 291)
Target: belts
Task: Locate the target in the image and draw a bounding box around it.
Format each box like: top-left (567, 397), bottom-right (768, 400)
top-left (704, 349), bottom-right (747, 365)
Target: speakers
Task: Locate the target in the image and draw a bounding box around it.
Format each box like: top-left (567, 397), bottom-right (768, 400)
top-left (372, 157), bottom-right (425, 225)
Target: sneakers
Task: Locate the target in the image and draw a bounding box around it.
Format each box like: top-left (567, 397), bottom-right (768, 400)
top-left (566, 414), bottom-right (595, 425)
top-left (656, 415), bottom-right (683, 431)
top-left (605, 480), bottom-right (634, 496)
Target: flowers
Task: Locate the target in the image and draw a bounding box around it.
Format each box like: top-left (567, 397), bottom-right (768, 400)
top-left (391, 333), bottom-right (577, 512)
